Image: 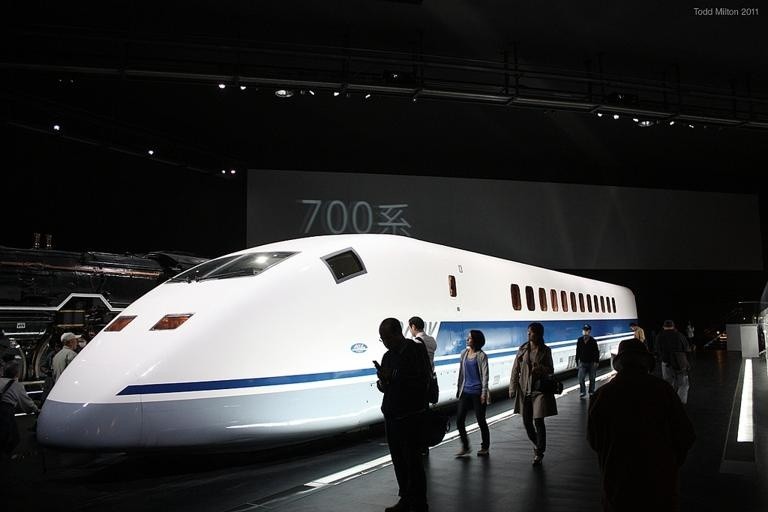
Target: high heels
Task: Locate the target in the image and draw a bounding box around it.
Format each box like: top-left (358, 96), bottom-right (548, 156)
top-left (531, 455), bottom-right (543, 465)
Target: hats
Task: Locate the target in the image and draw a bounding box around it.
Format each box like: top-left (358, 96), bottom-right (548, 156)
top-left (582, 325), bottom-right (591, 330)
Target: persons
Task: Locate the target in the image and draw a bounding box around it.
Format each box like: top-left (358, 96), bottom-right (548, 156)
top-left (585, 340), bottom-right (703, 512)
top-left (629, 319), bottom-right (695, 406)
top-left (0, 328), bottom-right (88, 463)
top-left (575, 325), bottom-right (600, 397)
top-left (373, 317), bottom-right (431, 512)
top-left (455, 330), bottom-right (491, 457)
top-left (408, 317), bottom-right (438, 373)
top-left (509, 321), bottom-right (558, 467)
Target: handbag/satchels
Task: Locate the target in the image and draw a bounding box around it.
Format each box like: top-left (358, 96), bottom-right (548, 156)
top-left (422, 410), bottom-right (450, 448)
top-left (429, 378), bottom-right (439, 404)
top-left (536, 377), bottom-right (564, 394)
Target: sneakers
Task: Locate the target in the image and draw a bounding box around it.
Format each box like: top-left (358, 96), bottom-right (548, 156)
top-left (477, 449), bottom-right (488, 455)
top-left (386, 500), bottom-right (428, 512)
top-left (454, 449), bottom-right (470, 456)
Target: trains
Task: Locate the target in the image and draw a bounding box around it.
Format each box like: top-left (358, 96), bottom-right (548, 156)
top-left (36, 228), bottom-right (637, 451)
top-left (0, 225), bottom-right (218, 408)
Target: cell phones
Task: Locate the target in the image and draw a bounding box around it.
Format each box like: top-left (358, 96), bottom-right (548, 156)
top-left (372, 360), bottom-right (381, 369)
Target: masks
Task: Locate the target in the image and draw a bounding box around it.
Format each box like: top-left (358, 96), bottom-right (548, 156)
top-left (582, 331), bottom-right (589, 335)
